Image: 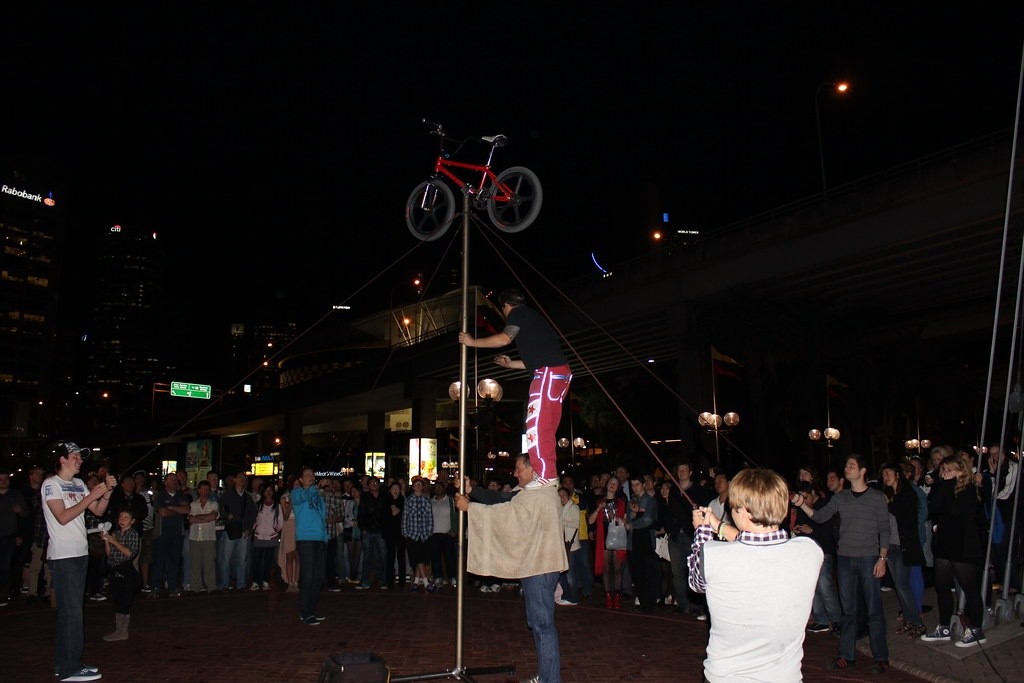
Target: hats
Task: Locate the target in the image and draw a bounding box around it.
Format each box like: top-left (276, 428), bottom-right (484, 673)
top-left (55, 442), bottom-right (90, 459)
top-left (411, 476), bottom-right (423, 481)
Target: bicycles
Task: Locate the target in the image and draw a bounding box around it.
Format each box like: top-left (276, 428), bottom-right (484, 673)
top-left (404, 117), bottom-right (544, 242)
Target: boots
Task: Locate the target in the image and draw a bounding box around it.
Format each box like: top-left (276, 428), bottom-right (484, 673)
top-left (605, 590), bottom-right (622, 611)
top-left (103, 612), bottom-right (130, 641)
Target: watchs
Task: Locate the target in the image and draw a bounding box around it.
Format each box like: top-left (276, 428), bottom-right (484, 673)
top-left (879, 555), bottom-right (889, 561)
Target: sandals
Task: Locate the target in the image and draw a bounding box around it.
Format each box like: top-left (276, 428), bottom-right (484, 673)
top-left (907, 623), bottom-right (927, 639)
top-left (895, 623), bottom-right (914, 634)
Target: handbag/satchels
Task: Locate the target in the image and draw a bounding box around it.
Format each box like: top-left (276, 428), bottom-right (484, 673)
top-left (605, 518), bottom-right (628, 550)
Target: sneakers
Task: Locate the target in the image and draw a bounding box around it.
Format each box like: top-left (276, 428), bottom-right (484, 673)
top-left (921, 624), bottom-right (951, 640)
top-left (827, 657), bottom-right (856, 669)
top-left (955, 627), bottom-right (986, 647)
top-left (54, 662), bottom-right (98, 676)
top-left (60, 666), bottom-right (101, 681)
top-left (868, 659), bottom-right (890, 673)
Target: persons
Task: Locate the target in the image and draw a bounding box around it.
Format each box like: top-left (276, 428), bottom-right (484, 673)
top-left (456, 453), bottom-right (570, 682)
top-left (100, 508), bottom-right (140, 642)
top-left (794, 454), bottom-right (893, 674)
top-left (459, 285), bottom-right (572, 489)
top-left (0, 444), bottom-right (1024, 646)
top-left (689, 469), bottom-right (825, 683)
top-left (40, 440), bottom-right (103, 682)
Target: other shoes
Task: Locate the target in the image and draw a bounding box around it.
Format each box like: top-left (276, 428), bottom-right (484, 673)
top-left (555, 599), bottom-right (578, 606)
top-left (300, 612), bottom-right (325, 620)
top-left (304, 615), bottom-right (320, 625)
top-left (806, 626), bottom-right (814, 631)
top-left (346, 573), bottom-right (523, 595)
top-left (814, 625), bottom-right (829, 632)
top-left (88, 578), bottom-right (309, 601)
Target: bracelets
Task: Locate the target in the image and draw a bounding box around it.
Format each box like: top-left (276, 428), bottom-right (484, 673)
top-left (719, 523), bottom-right (727, 540)
top-left (717, 521), bottom-right (724, 537)
